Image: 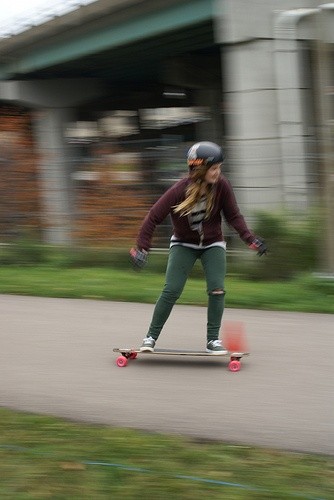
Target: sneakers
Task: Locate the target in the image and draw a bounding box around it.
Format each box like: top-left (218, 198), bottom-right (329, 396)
top-left (139, 336), bottom-right (155, 352)
top-left (206, 338), bottom-right (228, 354)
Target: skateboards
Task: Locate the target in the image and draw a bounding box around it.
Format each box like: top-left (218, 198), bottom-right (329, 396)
top-left (113, 347), bottom-right (251, 372)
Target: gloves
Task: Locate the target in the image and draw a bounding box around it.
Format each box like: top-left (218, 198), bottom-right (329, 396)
top-left (250, 238), bottom-right (270, 257)
top-left (130, 246), bottom-right (148, 269)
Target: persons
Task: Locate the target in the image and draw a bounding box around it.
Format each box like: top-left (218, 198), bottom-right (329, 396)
top-left (128, 141), bottom-right (272, 356)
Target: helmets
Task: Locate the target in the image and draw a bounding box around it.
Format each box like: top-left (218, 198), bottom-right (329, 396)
top-left (187, 141), bottom-right (224, 166)
